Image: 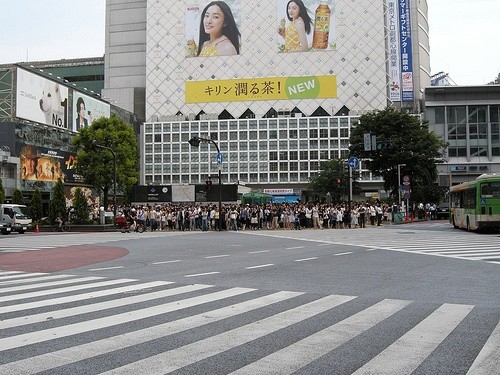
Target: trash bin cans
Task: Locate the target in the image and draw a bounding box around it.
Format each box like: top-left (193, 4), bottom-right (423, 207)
top-left (393, 208), bottom-right (403, 224)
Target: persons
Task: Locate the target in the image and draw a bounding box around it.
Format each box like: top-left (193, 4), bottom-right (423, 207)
top-left (186, 2), bottom-right (238, 57)
top-left (24, 145), bottom-right (40, 180)
top-left (76, 97), bottom-right (88, 132)
top-left (278, 0), bottom-right (313, 53)
top-left (56, 212), bottom-right (63, 228)
top-left (66, 198), bottom-right (437, 233)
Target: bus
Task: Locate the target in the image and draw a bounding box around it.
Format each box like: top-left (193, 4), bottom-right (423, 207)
top-left (444, 172), bottom-right (500, 233)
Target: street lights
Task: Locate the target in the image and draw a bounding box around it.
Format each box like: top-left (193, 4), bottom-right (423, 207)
top-left (93, 141), bottom-right (117, 230)
top-left (189, 135), bottom-right (224, 231)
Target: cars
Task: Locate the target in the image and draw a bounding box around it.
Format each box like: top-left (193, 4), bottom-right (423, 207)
top-left (0, 220), bottom-right (12, 235)
top-left (436, 202), bottom-right (450, 218)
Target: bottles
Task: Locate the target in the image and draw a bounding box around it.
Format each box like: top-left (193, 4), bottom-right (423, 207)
top-left (312, 0), bottom-right (331, 49)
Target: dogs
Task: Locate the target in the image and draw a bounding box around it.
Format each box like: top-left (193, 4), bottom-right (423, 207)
top-left (40, 83), bottom-right (64, 124)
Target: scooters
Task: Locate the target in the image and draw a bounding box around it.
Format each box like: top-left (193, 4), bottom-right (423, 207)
top-left (120, 220), bottom-right (145, 233)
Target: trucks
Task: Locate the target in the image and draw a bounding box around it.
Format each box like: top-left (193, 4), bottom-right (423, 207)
top-left (0, 204), bottom-right (32, 234)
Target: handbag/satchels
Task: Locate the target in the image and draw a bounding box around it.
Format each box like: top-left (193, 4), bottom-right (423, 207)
top-left (153, 221), bottom-right (158, 230)
